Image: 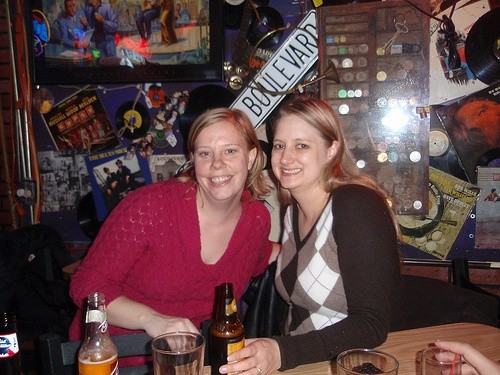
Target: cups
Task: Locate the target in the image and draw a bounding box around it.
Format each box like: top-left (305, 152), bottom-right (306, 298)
top-left (421, 346), bottom-right (462, 375)
top-left (336, 349), bottom-right (399, 375)
top-left (150, 332), bottom-right (205, 375)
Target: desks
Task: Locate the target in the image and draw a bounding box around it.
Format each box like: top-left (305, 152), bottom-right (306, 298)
top-left (201, 322), bottom-right (500, 375)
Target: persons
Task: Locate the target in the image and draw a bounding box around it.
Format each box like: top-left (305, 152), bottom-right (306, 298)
top-left (64, 118), bottom-right (104, 149)
top-left (69, 108), bottom-right (280, 351)
top-left (484, 189), bottom-right (500, 201)
top-left (434, 341), bottom-right (500, 375)
top-left (78, 0), bottom-right (118, 59)
top-left (57, 0), bottom-right (91, 54)
top-left (136, 0), bottom-right (160, 48)
top-left (219, 95), bottom-right (404, 375)
top-left (116, 160), bottom-right (131, 191)
top-left (103, 167), bottom-right (121, 211)
top-left (151, 0), bottom-right (178, 46)
top-left (452, 100), bottom-right (500, 167)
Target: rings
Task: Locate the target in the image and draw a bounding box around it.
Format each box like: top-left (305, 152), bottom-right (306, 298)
top-left (257, 368), bottom-right (263, 374)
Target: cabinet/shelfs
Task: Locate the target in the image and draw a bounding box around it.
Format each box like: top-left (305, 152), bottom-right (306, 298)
top-left (317, 0), bottom-right (430, 215)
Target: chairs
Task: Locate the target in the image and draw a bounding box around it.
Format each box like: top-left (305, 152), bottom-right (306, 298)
top-left (38, 320), bottom-right (211, 375)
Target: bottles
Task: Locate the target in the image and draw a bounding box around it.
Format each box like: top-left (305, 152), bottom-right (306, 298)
top-left (210, 282), bottom-right (245, 375)
top-left (78, 292), bottom-right (119, 375)
top-left (0, 311), bottom-right (22, 375)
